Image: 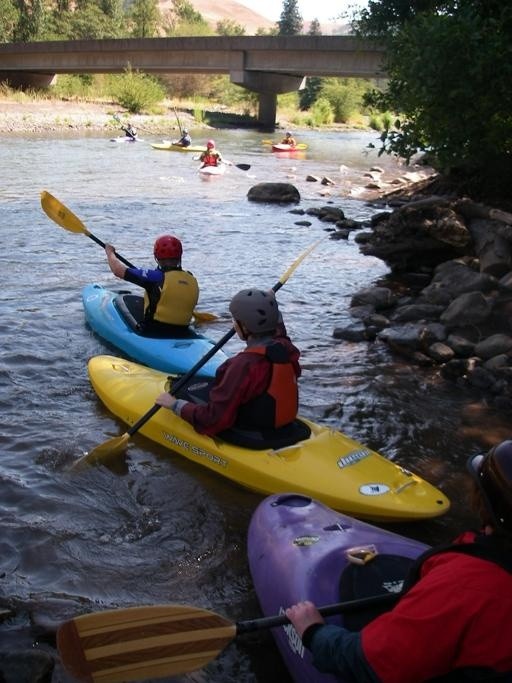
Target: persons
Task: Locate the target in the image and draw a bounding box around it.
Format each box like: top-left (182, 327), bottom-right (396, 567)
top-left (171, 129), bottom-right (192, 147)
top-left (192, 140), bottom-right (228, 169)
top-left (277, 131), bottom-right (296, 148)
top-left (105, 234), bottom-right (200, 339)
top-left (121, 123), bottom-right (137, 137)
top-left (284, 436), bottom-right (512, 683)
top-left (155, 288), bottom-right (302, 450)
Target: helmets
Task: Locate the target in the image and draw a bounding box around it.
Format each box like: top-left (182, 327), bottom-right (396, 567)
top-left (183, 129), bottom-right (293, 149)
top-left (229, 289), bottom-right (278, 333)
top-left (154, 235), bottom-right (182, 258)
top-left (467, 438), bottom-right (512, 528)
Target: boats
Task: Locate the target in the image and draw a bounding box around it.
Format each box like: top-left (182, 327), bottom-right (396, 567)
top-left (110, 134), bottom-right (138, 143)
top-left (274, 151), bottom-right (308, 160)
top-left (87, 354), bottom-right (452, 521)
top-left (82, 281), bottom-right (230, 376)
top-left (149, 141), bottom-right (207, 151)
top-left (197, 158), bottom-right (227, 174)
top-left (247, 490), bottom-right (436, 682)
top-left (271, 142), bottom-right (309, 151)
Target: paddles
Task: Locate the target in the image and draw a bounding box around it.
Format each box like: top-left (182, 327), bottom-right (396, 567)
top-left (58, 593), bottom-right (400, 681)
top-left (41, 191), bottom-right (219, 325)
top-left (113, 115), bottom-right (136, 140)
top-left (70, 230), bottom-right (336, 468)
top-left (233, 163), bottom-right (251, 171)
